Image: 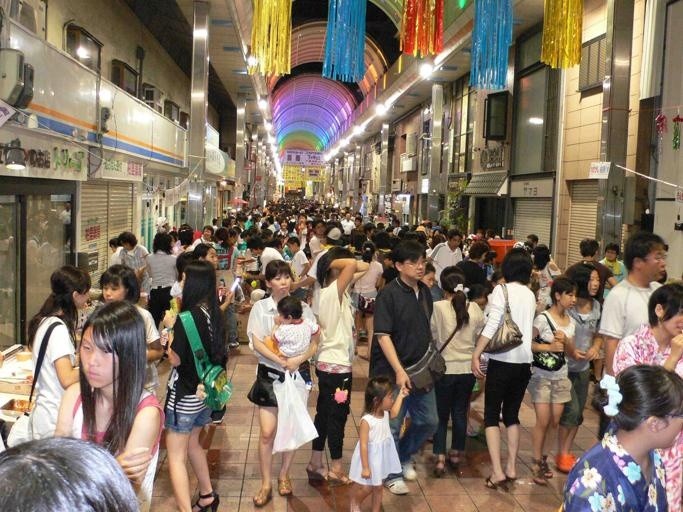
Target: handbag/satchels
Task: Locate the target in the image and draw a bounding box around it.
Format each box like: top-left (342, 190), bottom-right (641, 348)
top-left (532, 335), bottom-right (565, 371)
top-left (474, 319), bottom-right (523, 354)
top-left (404, 342), bottom-right (446, 396)
top-left (202, 365), bottom-right (234, 411)
top-left (7, 412), bottom-right (33, 449)
top-left (358, 294), bottom-right (376, 313)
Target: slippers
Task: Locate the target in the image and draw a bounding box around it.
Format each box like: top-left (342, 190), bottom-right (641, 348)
top-left (505, 473), bottom-right (518, 485)
top-left (485, 473), bottom-right (509, 492)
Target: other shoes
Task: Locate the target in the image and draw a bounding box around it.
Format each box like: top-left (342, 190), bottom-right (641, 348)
top-left (554, 454), bottom-right (576, 472)
top-left (349, 498), bottom-right (361, 512)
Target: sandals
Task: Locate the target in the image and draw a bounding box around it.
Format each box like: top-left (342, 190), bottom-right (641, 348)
top-left (278, 475), bottom-right (293, 496)
top-left (539, 454), bottom-right (553, 478)
top-left (528, 457), bottom-right (547, 485)
top-left (252, 487), bottom-right (272, 507)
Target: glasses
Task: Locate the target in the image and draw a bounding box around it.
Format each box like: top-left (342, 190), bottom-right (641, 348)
top-left (401, 260), bottom-right (429, 268)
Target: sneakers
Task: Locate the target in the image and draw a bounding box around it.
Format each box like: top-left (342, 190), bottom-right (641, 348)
top-left (386, 477), bottom-right (410, 494)
top-left (401, 462), bottom-right (417, 480)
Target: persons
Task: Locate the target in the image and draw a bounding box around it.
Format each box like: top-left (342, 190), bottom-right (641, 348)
top-left (1, 200), bottom-right (683, 510)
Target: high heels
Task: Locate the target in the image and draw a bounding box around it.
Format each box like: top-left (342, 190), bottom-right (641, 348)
top-left (449, 454), bottom-right (460, 472)
top-left (193, 489), bottom-right (220, 512)
top-left (433, 461), bottom-right (446, 478)
top-left (306, 463), bottom-right (328, 482)
top-left (327, 471), bottom-right (355, 487)
